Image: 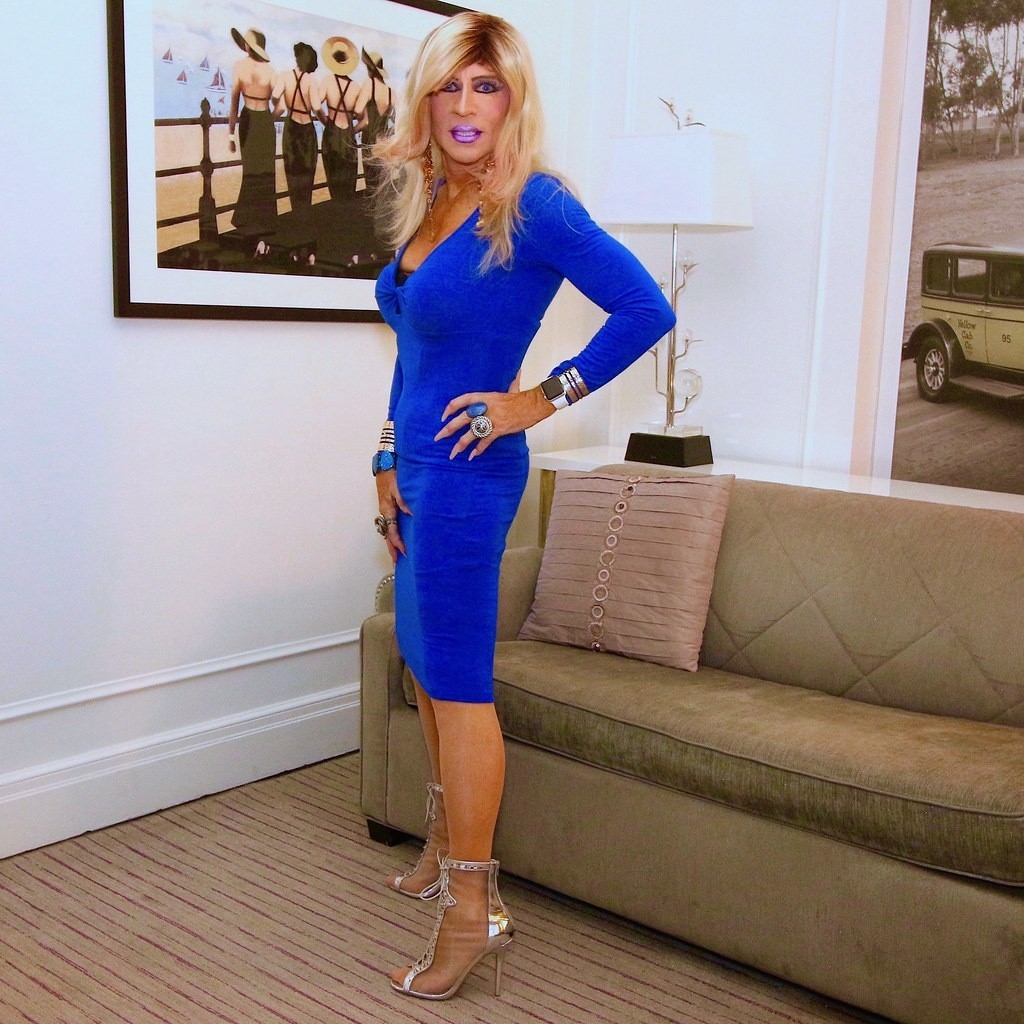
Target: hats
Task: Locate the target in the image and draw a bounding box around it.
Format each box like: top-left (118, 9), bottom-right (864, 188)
top-left (321, 37), bottom-right (359, 76)
top-left (231, 28), bottom-right (270, 62)
top-left (361, 47), bottom-right (390, 78)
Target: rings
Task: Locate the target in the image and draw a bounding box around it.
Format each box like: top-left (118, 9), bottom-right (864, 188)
top-left (471, 416), bottom-right (494, 438)
top-left (374, 514), bottom-right (397, 536)
top-left (466, 402), bottom-right (488, 417)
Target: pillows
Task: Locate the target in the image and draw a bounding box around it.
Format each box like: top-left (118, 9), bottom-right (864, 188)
top-left (516, 468), bottom-right (737, 675)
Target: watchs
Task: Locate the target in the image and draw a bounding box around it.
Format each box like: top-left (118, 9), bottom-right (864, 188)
top-left (540, 375), bottom-right (570, 410)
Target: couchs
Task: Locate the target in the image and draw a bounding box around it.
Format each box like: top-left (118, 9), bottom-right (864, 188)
top-left (354, 451), bottom-right (1024, 1024)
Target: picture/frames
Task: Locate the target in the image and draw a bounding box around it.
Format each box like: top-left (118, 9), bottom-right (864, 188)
top-left (105, 0), bottom-right (485, 325)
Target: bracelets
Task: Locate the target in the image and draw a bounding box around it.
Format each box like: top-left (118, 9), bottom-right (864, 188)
top-left (372, 450), bottom-right (395, 476)
top-left (230, 134), bottom-right (236, 141)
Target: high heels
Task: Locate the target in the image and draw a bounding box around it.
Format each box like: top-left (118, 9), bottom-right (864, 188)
top-left (254, 242), bottom-right (270, 261)
top-left (306, 255), bottom-right (315, 267)
top-left (390, 857), bottom-right (516, 1000)
top-left (389, 784), bottom-right (450, 901)
top-left (346, 254), bottom-right (359, 268)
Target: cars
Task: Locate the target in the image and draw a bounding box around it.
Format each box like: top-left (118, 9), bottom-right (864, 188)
top-left (901, 242), bottom-right (1024, 403)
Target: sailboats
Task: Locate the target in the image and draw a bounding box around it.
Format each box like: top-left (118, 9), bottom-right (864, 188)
top-left (205, 68), bottom-right (226, 93)
top-left (211, 96), bottom-right (229, 116)
top-left (199, 57), bottom-right (210, 73)
top-left (161, 47), bottom-right (173, 63)
top-left (176, 70), bottom-right (188, 85)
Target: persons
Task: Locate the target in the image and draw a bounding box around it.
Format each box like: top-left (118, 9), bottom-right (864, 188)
top-left (271, 37), bottom-right (398, 273)
top-left (376, 11), bottom-right (677, 998)
top-left (228, 27), bottom-right (285, 264)
